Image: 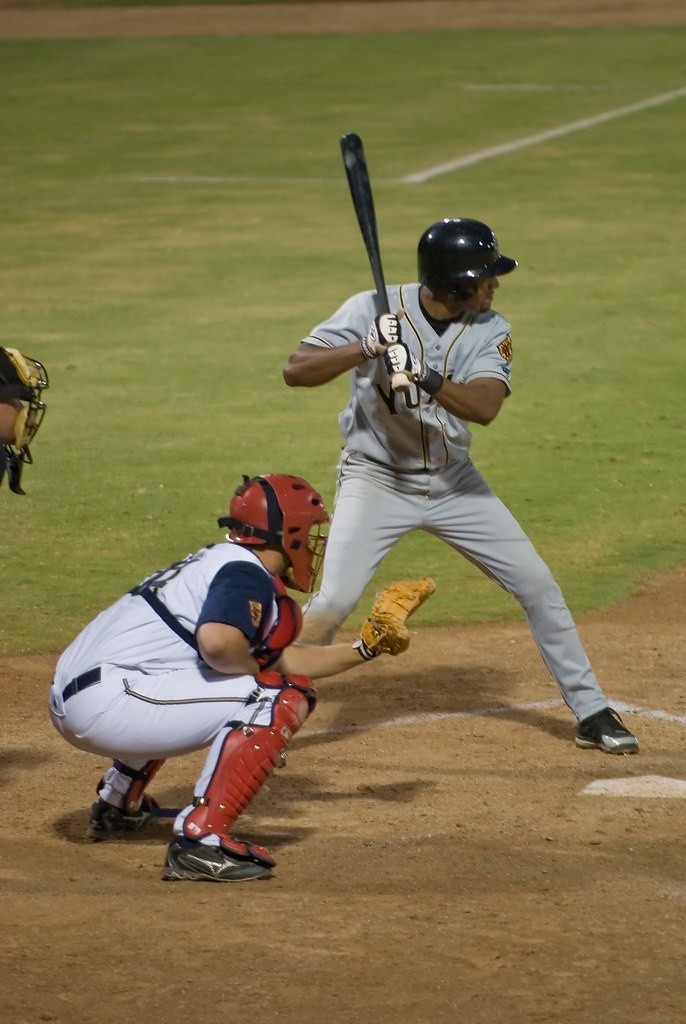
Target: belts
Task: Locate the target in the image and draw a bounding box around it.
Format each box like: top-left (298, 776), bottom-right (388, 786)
top-left (366, 455), bottom-right (429, 474)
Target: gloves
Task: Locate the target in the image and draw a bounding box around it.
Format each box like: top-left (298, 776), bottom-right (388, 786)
top-left (383, 343), bottom-right (443, 395)
top-left (360, 308), bottom-right (405, 360)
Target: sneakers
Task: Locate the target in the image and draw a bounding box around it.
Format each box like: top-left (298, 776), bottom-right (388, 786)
top-left (163, 835), bottom-right (271, 881)
top-left (575, 706), bottom-right (640, 754)
top-left (87, 802), bottom-right (155, 839)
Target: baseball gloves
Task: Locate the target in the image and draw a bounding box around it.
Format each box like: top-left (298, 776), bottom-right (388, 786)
top-left (359, 577), bottom-right (438, 652)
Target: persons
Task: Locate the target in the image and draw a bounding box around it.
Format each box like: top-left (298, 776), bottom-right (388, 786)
top-left (0, 346), bottom-right (49, 496)
top-left (48, 470), bottom-right (436, 883)
top-left (283, 216), bottom-right (638, 754)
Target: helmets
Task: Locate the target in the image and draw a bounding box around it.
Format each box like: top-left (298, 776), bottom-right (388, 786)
top-left (218, 473), bottom-right (331, 593)
top-left (0, 346), bottom-right (37, 402)
top-left (417, 217), bottom-right (517, 302)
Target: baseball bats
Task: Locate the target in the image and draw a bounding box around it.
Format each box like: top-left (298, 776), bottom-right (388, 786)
top-left (339, 127), bottom-right (409, 396)
